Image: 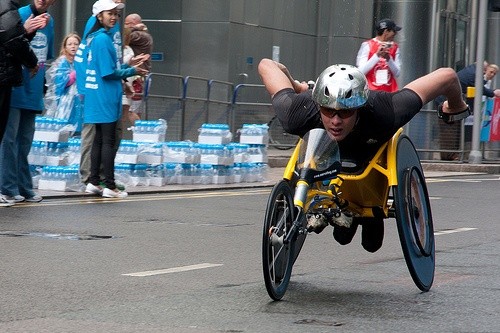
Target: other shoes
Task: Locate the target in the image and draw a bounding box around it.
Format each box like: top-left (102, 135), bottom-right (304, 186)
top-left (102, 187), bottom-right (128, 197)
top-left (0, 192), bottom-right (25, 203)
top-left (85, 182), bottom-right (103, 196)
top-left (24, 195), bottom-right (43, 202)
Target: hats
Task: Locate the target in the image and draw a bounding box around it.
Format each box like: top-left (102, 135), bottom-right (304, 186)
top-left (92, 0), bottom-right (125, 17)
top-left (375, 19), bottom-right (401, 32)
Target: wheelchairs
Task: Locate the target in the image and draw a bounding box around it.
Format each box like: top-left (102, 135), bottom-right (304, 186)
top-left (262, 124), bottom-right (436, 302)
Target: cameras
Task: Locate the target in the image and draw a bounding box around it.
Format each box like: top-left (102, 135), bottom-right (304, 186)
top-left (385, 43), bottom-right (393, 48)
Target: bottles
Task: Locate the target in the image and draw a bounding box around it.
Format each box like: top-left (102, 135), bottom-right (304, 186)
top-left (26, 115), bottom-right (82, 180)
top-left (113, 119), bottom-right (268, 178)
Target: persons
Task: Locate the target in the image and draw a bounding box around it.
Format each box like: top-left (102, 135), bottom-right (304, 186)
top-left (0, 0), bottom-right (55, 203)
top-left (437, 59), bottom-right (500, 160)
top-left (72, 0), bottom-right (153, 198)
top-left (42, 31), bottom-right (81, 139)
top-left (257, 57), bottom-right (472, 254)
top-left (355, 18), bottom-right (403, 93)
top-left (0, 0), bottom-right (50, 207)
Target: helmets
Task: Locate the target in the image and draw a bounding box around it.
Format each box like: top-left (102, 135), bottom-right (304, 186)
top-left (311, 63), bottom-right (371, 110)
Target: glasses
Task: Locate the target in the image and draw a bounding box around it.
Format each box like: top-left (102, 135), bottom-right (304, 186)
top-left (319, 105), bottom-right (355, 119)
